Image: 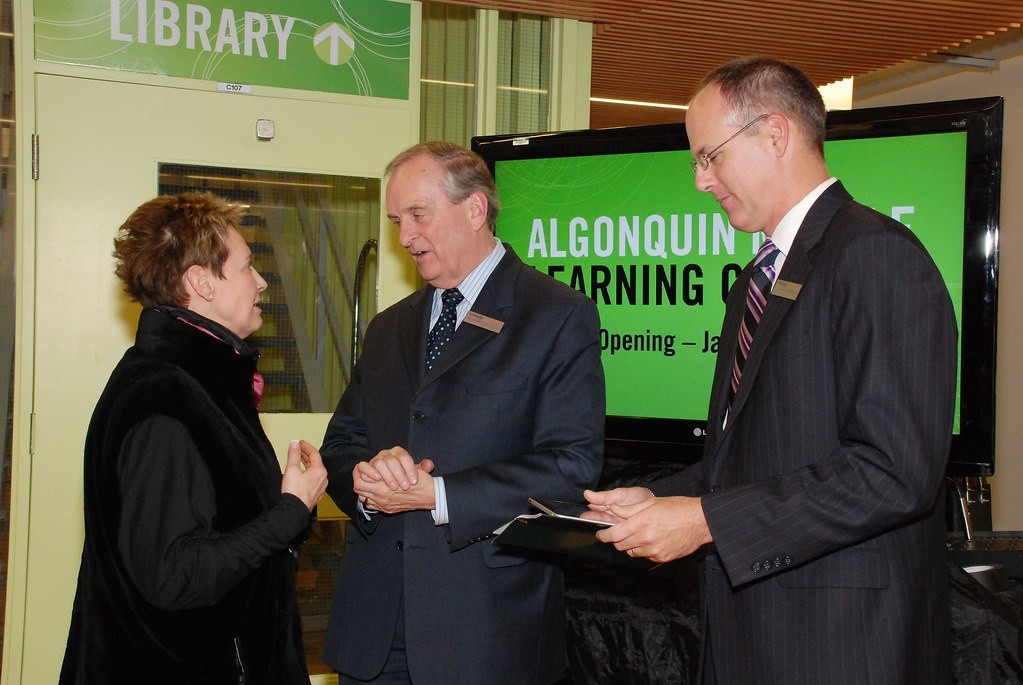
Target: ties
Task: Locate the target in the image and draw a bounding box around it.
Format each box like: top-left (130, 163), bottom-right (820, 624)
top-left (426, 288), bottom-right (464, 377)
top-left (727, 238), bottom-right (780, 419)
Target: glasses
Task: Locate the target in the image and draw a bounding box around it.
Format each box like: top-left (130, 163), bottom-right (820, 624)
top-left (690, 113), bottom-right (769, 175)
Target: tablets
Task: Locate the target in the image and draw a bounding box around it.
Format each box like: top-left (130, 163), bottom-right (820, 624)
top-left (528, 497), bottom-right (620, 525)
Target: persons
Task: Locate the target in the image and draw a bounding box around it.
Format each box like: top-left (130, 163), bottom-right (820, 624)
top-left (58, 194), bottom-right (329, 685)
top-left (320, 142), bottom-right (605, 685)
top-left (584, 57), bottom-right (959, 685)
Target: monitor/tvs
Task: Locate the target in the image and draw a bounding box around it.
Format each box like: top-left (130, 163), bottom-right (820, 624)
top-left (472, 95), bottom-right (1005, 477)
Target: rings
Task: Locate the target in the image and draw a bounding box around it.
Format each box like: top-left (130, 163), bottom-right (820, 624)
top-left (365, 497), bottom-right (370, 505)
top-left (632, 547), bottom-right (639, 558)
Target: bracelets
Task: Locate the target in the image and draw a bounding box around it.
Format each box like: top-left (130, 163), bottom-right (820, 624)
top-left (643, 487), bottom-right (655, 498)
